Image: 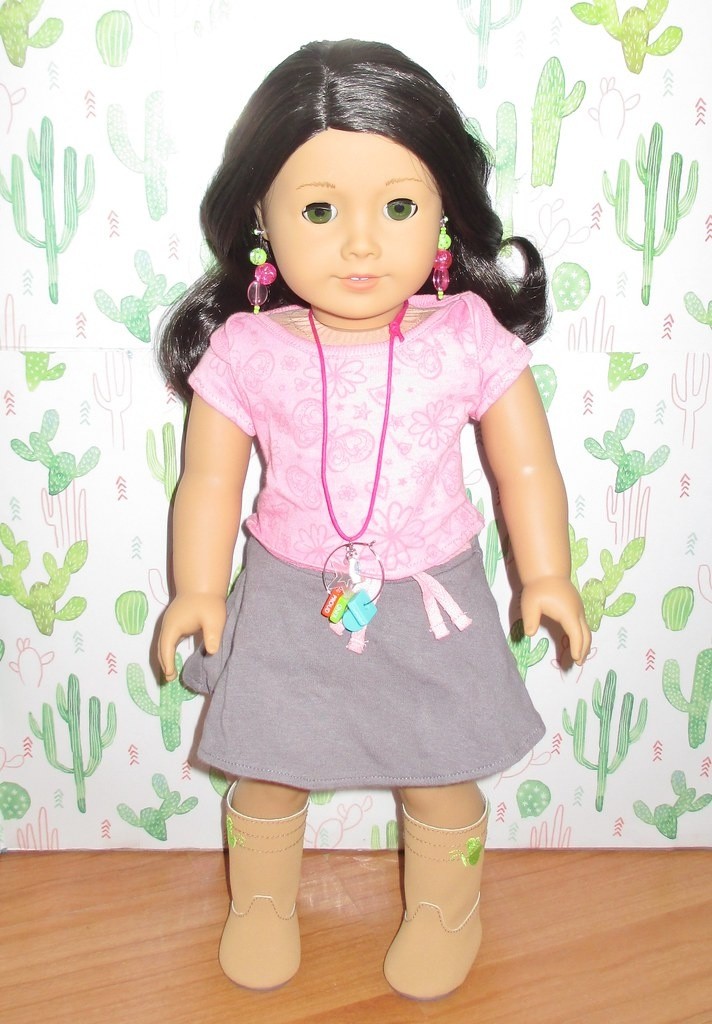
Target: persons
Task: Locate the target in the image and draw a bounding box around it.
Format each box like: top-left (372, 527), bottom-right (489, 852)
top-left (157, 38), bottom-right (592, 999)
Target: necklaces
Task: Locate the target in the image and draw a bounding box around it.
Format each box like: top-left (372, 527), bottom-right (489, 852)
top-left (308, 301), bottom-right (409, 631)
